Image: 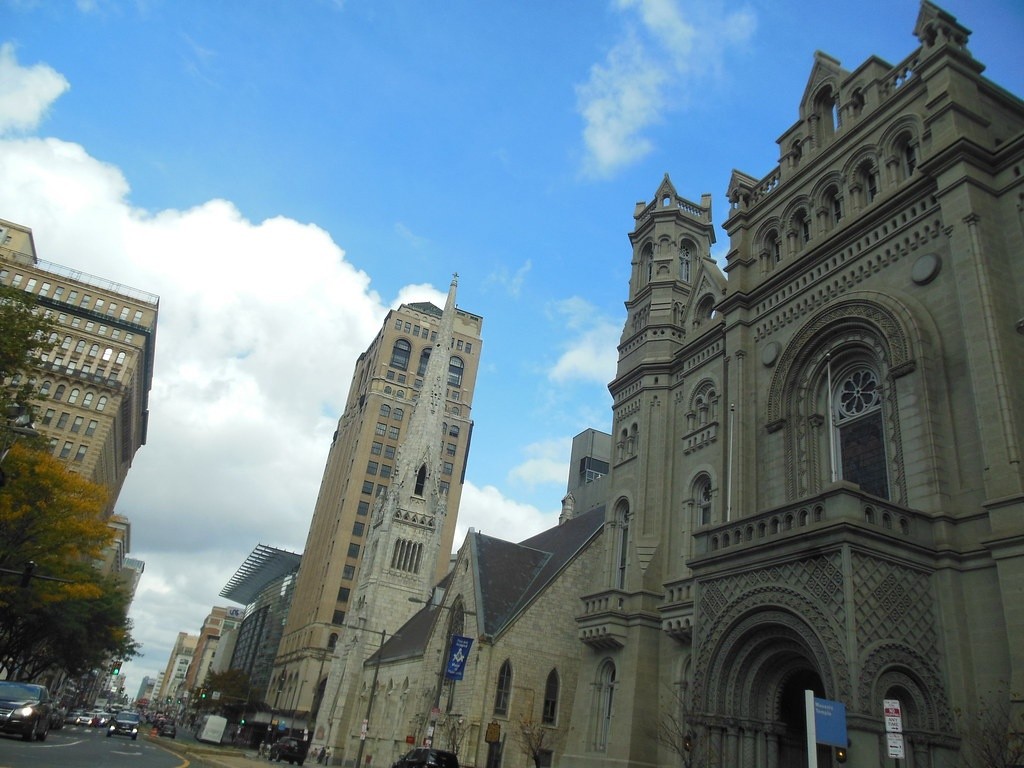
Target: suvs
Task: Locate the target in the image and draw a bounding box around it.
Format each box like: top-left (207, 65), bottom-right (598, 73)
top-left (268, 737), bottom-right (308, 766)
top-left (391, 748), bottom-right (459, 768)
top-left (106, 711), bottom-right (139, 740)
top-left (0, 681), bottom-right (51, 741)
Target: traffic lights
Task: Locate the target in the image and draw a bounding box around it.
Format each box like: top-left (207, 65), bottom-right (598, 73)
top-left (199, 688), bottom-right (208, 704)
top-left (177, 698), bottom-right (181, 704)
top-left (167, 697), bottom-right (172, 704)
top-left (241, 719), bottom-right (246, 726)
top-left (111, 661), bottom-right (123, 676)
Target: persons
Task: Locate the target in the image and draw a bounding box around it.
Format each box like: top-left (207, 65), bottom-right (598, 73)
top-left (309, 747), bottom-right (333, 767)
top-left (259, 740), bottom-right (272, 760)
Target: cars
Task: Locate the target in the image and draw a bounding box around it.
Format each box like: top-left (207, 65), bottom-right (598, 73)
top-left (64, 709), bottom-right (111, 726)
top-left (140, 711), bottom-right (176, 739)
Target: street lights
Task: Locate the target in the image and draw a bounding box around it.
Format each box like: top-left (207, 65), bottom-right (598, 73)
top-left (347, 625), bottom-right (403, 768)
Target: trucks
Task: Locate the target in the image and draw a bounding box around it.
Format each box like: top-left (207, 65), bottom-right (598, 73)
top-left (194, 714), bottom-right (228, 745)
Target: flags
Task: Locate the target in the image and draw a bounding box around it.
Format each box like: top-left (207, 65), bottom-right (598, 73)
top-left (445, 634), bottom-right (475, 681)
